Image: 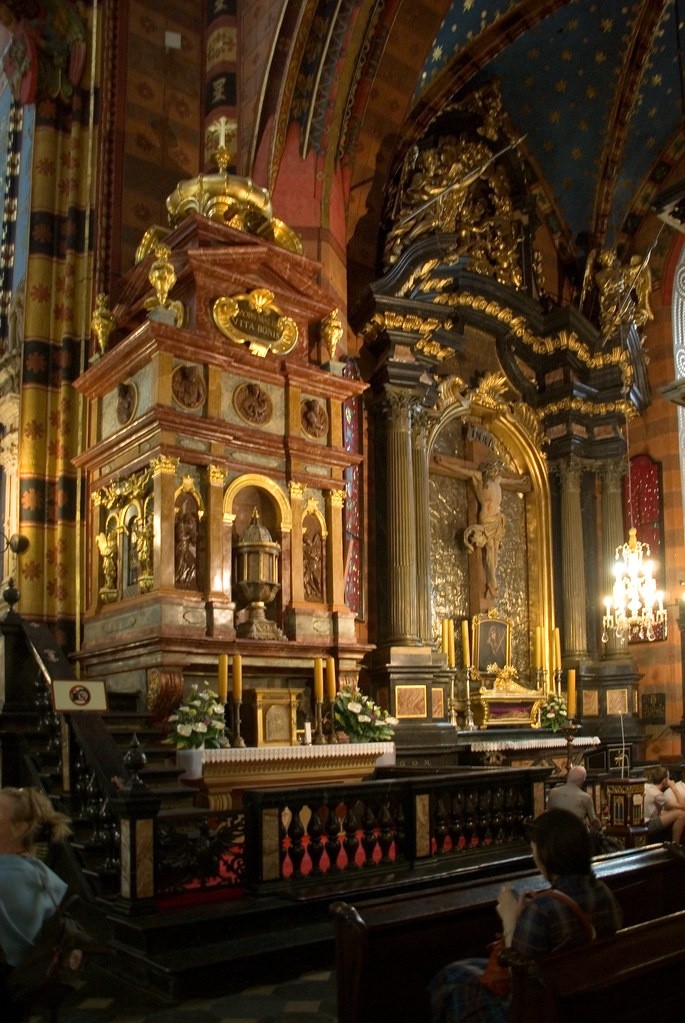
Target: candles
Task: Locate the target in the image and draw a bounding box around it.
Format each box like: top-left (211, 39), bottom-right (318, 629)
top-left (326, 656), bottom-right (336, 701)
top-left (314, 657), bottom-right (324, 704)
top-left (233, 655), bottom-right (243, 704)
top-left (219, 654), bottom-right (228, 704)
top-left (554, 627), bottom-right (562, 668)
top-left (442, 618), bottom-right (455, 668)
top-left (567, 668), bottom-right (577, 720)
top-left (461, 619), bottom-right (470, 668)
top-left (535, 626), bottom-right (541, 669)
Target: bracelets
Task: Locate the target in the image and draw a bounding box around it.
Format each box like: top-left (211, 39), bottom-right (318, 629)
top-left (503, 927), bottom-right (512, 938)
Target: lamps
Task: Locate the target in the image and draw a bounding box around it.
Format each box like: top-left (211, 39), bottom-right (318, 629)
top-left (601, 176), bottom-right (669, 648)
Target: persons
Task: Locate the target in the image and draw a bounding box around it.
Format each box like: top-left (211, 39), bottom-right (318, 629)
top-left (661, 763), bottom-right (685, 818)
top-left (640, 764), bottom-right (685, 858)
top-left (546, 766), bottom-right (603, 833)
top-left (434, 807), bottom-right (620, 1022)
top-left (435, 455), bottom-right (530, 599)
top-left (0, 784), bottom-right (109, 1023)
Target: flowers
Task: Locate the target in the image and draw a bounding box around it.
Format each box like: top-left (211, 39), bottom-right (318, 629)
top-left (324, 675), bottom-right (399, 743)
top-left (159, 680), bottom-right (234, 751)
top-left (538, 692), bottom-right (569, 735)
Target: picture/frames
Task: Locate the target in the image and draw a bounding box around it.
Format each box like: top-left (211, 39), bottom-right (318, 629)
top-left (467, 607), bottom-right (514, 681)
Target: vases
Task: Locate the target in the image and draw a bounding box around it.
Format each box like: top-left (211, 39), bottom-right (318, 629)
top-left (176, 746), bottom-right (203, 781)
top-left (373, 741), bottom-right (397, 767)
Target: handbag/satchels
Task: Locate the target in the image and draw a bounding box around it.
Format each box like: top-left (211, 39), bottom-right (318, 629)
top-left (479, 890), bottom-right (593, 1001)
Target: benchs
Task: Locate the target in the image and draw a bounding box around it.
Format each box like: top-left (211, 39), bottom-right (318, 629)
top-left (508, 907), bottom-right (685, 1023)
